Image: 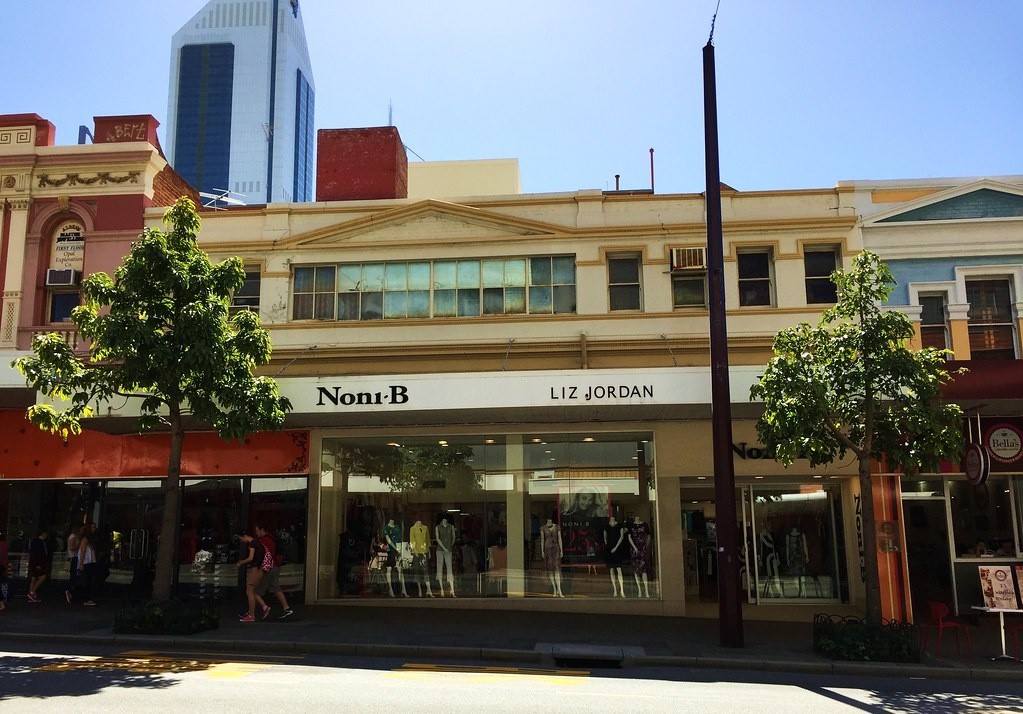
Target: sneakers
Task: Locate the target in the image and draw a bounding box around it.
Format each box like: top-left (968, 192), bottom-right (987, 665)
top-left (276, 608), bottom-right (294, 620)
top-left (261, 606), bottom-right (271, 622)
top-left (26, 592), bottom-right (42, 603)
top-left (239, 615), bottom-right (256, 622)
top-left (83, 601), bottom-right (96, 606)
top-left (65, 590), bottom-right (72, 605)
top-left (239, 610), bottom-right (250, 618)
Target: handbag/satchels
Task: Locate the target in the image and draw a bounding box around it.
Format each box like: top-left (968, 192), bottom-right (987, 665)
top-left (260, 550), bottom-right (274, 573)
top-left (29, 557), bottom-right (42, 577)
top-left (80, 564), bottom-right (98, 575)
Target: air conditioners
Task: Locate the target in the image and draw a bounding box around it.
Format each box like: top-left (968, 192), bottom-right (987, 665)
top-left (672, 247), bottom-right (708, 271)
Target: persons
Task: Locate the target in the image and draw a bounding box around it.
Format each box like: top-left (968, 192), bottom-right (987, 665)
top-left (237, 528), bottom-right (273, 622)
top-left (628, 517), bottom-right (651, 598)
top-left (27, 528), bottom-right (52, 603)
top-left (760, 523), bottom-right (784, 598)
top-left (785, 528), bottom-right (810, 597)
top-left (560, 486), bottom-right (608, 517)
top-left (64, 520), bottom-right (113, 606)
top-left (382, 519), bottom-right (409, 598)
top-left (541, 519), bottom-right (565, 597)
top-left (239, 522), bottom-right (294, 618)
top-left (409, 520), bottom-right (435, 598)
top-left (435, 519), bottom-right (458, 597)
top-left (586, 534), bottom-right (599, 575)
top-left (0, 531), bottom-right (9, 611)
top-left (962, 539), bottom-right (1015, 557)
top-left (603, 517), bottom-right (627, 598)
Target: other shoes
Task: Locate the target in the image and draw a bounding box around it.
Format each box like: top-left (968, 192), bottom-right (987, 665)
top-left (0, 603), bottom-right (8, 615)
